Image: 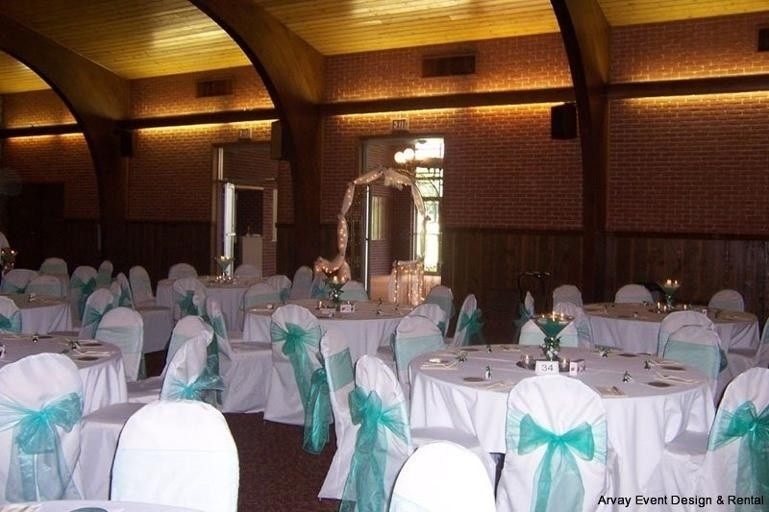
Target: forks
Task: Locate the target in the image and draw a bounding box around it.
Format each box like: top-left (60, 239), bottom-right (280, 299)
top-left (72, 349), bottom-right (109, 354)
top-left (423, 341), bottom-right (694, 397)
